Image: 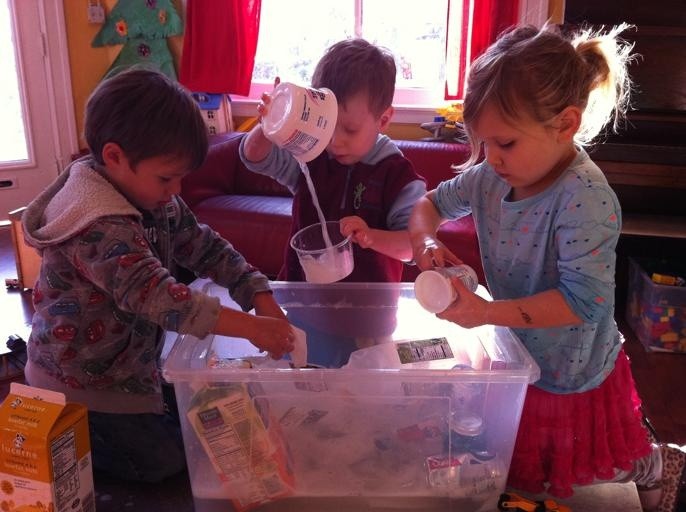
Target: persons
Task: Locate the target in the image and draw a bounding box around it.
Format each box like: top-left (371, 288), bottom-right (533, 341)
top-left (19, 70), bottom-right (297, 485)
top-left (409, 22), bottom-right (663, 512)
top-left (237, 39), bottom-right (429, 370)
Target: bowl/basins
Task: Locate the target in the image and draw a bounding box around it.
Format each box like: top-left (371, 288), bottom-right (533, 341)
top-left (260, 81), bottom-right (339, 163)
top-left (290, 220), bottom-right (355, 285)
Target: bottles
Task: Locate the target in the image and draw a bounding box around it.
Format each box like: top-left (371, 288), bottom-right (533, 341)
top-left (444, 409), bottom-right (485, 455)
top-left (373, 416), bottom-right (450, 451)
top-left (334, 335), bottom-right (471, 396)
top-left (240, 358), bottom-right (350, 430)
top-left (425, 448), bottom-right (505, 490)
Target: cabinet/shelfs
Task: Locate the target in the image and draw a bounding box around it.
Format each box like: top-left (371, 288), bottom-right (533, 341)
top-left (582, 120), bottom-right (686, 239)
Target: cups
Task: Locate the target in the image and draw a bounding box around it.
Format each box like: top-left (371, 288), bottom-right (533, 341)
top-left (414, 264), bottom-right (479, 315)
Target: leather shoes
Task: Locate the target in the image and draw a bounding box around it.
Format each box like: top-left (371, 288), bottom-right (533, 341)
top-left (639, 445), bottom-right (686, 511)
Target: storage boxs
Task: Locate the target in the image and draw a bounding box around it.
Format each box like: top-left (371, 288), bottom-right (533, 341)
top-left (163, 276), bottom-right (541, 512)
top-left (626, 261), bottom-right (685, 353)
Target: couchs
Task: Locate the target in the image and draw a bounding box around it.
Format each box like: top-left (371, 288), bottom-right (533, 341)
top-left (180, 133), bottom-right (485, 285)
